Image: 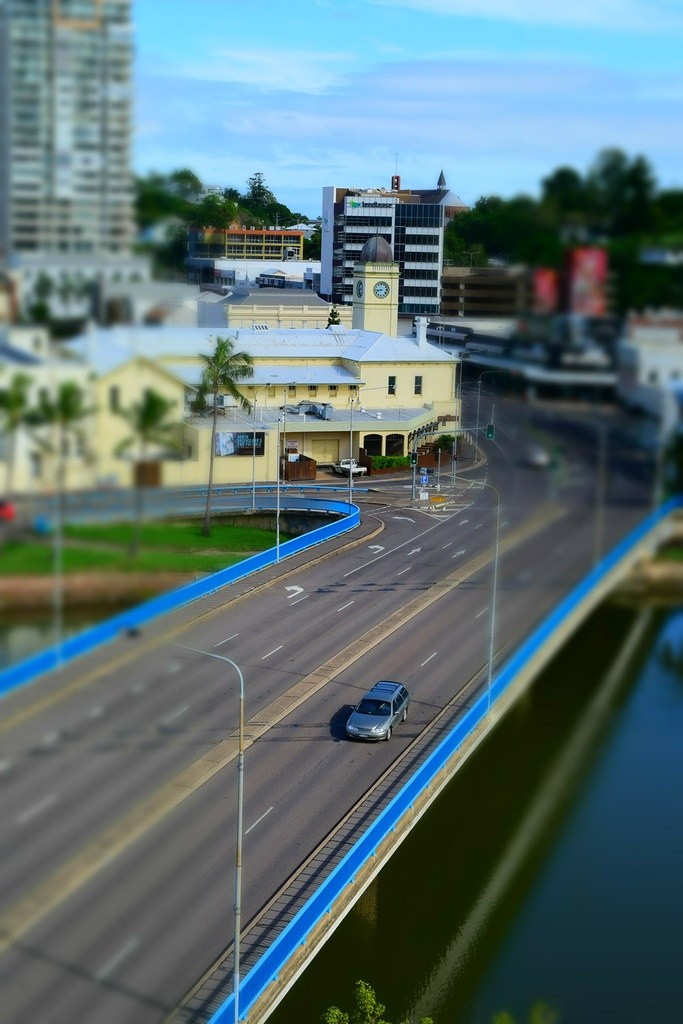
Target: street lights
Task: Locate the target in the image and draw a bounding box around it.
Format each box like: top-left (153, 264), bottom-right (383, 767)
top-left (463, 251), bottom-right (481, 268)
top-left (252, 383), bottom-right (272, 510)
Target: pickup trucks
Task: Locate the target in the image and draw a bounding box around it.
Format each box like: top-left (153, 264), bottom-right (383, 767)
top-left (333, 459), bottom-right (367, 478)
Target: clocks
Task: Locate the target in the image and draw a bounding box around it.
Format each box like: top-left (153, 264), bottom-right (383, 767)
top-left (373, 281), bottom-right (390, 299)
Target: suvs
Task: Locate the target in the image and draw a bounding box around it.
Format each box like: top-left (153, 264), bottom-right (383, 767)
top-left (345, 681), bottom-right (410, 741)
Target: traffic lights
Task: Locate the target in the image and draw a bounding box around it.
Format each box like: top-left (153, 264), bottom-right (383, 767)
top-left (487, 425), bottom-right (493, 438)
top-left (412, 453), bottom-right (417, 464)
top-left (433, 451), bottom-right (438, 461)
